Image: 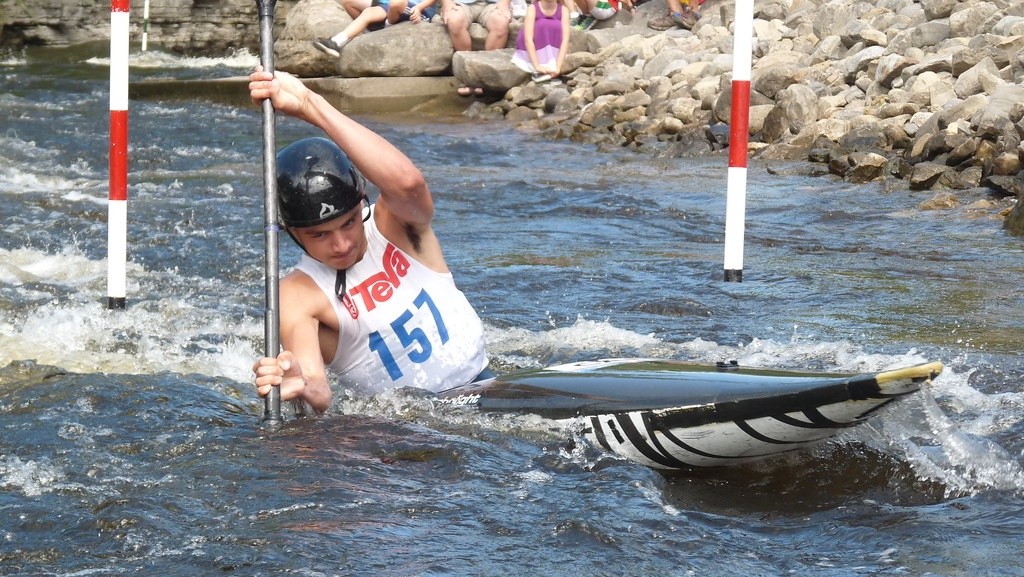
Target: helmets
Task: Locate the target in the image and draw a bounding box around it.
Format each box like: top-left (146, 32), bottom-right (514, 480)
top-left (275, 137), bottom-right (367, 227)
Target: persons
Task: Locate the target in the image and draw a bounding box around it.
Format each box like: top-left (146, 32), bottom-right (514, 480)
top-left (312, 0), bottom-right (706, 97)
top-left (248, 65), bottom-right (498, 413)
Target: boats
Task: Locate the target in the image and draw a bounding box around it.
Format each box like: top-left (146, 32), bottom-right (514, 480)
top-left (435, 355), bottom-right (941, 479)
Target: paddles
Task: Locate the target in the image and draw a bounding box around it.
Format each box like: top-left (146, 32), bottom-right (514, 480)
top-left (254, 0), bottom-right (287, 434)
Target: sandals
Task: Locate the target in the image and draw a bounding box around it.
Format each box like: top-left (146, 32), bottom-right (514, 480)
top-left (457, 82), bottom-right (483, 98)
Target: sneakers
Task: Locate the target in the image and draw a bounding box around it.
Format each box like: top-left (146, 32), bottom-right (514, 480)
top-left (369, 22), bottom-right (386, 31)
top-left (313, 35), bottom-right (341, 58)
top-left (571, 14), bottom-right (586, 26)
top-left (673, 9), bottom-right (701, 30)
top-left (647, 10), bottom-right (680, 31)
top-left (579, 15), bottom-right (598, 32)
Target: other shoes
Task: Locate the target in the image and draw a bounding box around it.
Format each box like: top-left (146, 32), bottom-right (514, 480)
top-left (531, 73), bottom-right (551, 83)
